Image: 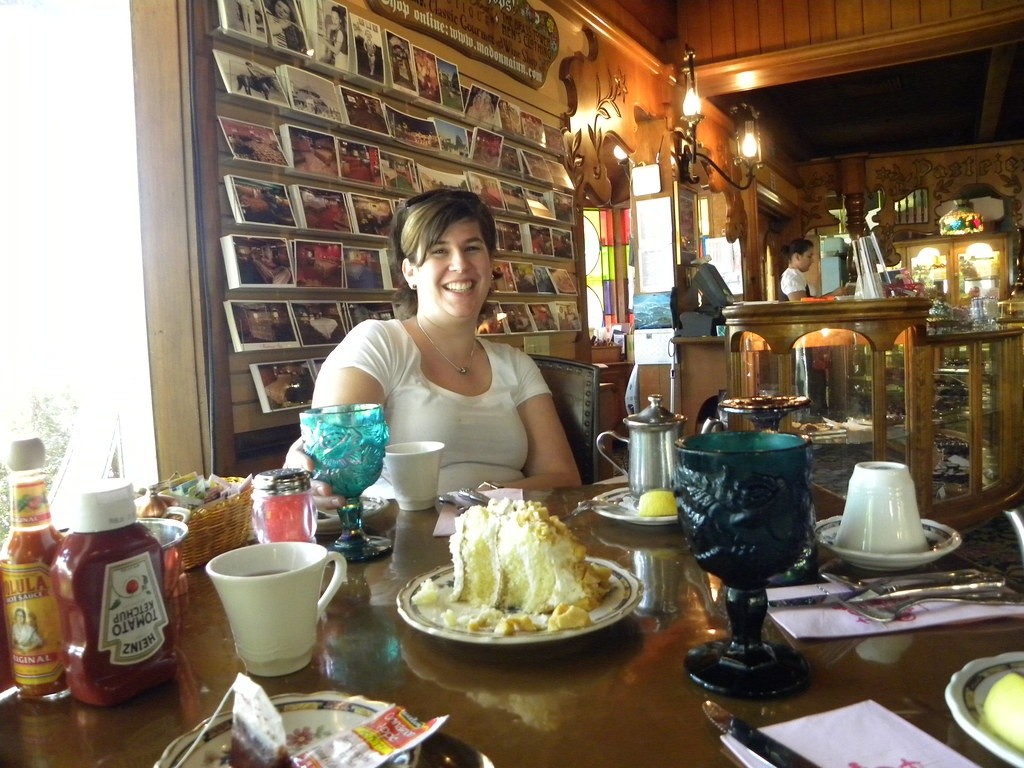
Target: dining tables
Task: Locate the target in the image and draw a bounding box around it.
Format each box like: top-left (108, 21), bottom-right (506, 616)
top-left (0, 438), bottom-right (1024, 768)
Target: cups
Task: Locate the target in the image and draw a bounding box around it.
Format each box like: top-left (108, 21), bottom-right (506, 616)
top-left (380, 441), bottom-right (446, 510)
top-left (835, 461), bottom-right (931, 554)
top-left (971, 297), bottom-right (1002, 332)
top-left (204, 541), bottom-right (346, 678)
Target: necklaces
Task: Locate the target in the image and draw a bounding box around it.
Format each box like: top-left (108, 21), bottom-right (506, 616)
top-left (417, 319), bottom-right (477, 374)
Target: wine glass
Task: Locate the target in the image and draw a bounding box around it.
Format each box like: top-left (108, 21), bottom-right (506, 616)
top-left (672, 432), bottom-right (818, 697)
top-left (301, 404), bottom-right (394, 559)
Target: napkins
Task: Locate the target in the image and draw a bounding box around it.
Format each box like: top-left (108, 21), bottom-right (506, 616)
top-left (765, 580), bottom-right (1024, 640)
top-left (433, 487), bottom-right (523, 537)
top-left (721, 699), bottom-right (981, 768)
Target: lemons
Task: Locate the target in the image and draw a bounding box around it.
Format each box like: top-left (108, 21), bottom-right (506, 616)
top-left (637, 490), bottom-right (678, 515)
top-left (982, 672), bottom-right (1024, 751)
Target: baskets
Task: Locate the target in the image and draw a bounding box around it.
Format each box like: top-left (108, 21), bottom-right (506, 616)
top-left (168, 476), bottom-right (255, 572)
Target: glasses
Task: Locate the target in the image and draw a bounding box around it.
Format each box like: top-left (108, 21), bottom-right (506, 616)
top-left (404, 188), bottom-right (478, 210)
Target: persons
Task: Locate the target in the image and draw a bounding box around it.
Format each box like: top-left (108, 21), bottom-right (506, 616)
top-left (237, 0), bottom-right (349, 70)
top-left (245, 61), bottom-right (262, 91)
top-left (778, 238), bottom-right (848, 301)
top-left (532, 230), bottom-right (545, 254)
top-left (364, 30), bottom-right (377, 75)
top-left (287, 189), bottom-right (581, 512)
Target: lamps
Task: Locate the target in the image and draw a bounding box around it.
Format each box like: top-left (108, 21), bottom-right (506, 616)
top-left (937, 198), bottom-right (982, 235)
top-left (668, 46), bottom-right (762, 189)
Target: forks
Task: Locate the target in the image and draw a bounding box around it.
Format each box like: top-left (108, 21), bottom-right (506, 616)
top-left (817, 586), bottom-right (1024, 623)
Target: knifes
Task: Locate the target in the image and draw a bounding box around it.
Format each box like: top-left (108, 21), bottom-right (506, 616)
top-left (765, 574), bottom-right (1004, 608)
top-left (702, 700), bottom-right (822, 768)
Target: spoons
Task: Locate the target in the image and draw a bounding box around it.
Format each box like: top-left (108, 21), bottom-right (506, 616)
top-left (822, 571), bottom-right (984, 591)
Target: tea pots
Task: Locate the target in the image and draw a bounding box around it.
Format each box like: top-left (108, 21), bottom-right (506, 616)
top-left (595, 394), bottom-right (719, 510)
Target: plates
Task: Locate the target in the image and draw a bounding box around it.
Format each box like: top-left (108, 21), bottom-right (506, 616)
top-left (945, 651), bottom-right (1024, 768)
top-left (153, 691), bottom-right (421, 768)
top-left (398, 555), bottom-right (646, 645)
top-left (817, 516), bottom-right (961, 568)
top-left (588, 487), bottom-right (681, 525)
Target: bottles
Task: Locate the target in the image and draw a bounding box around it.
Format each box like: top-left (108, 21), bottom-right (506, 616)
top-left (2, 438), bottom-right (173, 704)
top-left (250, 468), bottom-right (316, 546)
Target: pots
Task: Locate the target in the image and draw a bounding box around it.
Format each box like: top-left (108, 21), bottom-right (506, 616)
top-left (136, 507), bottom-right (191, 609)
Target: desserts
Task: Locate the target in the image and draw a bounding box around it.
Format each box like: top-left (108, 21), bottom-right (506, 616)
top-left (447, 498), bottom-right (616, 632)
